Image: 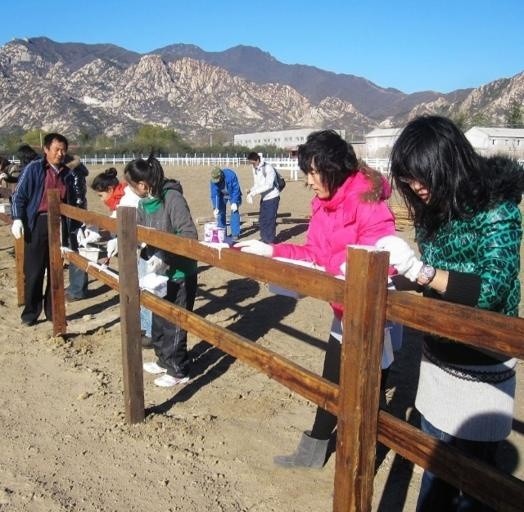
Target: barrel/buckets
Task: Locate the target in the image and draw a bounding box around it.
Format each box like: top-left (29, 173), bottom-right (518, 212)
top-left (226, 219), bottom-right (232, 237)
top-left (208, 227), bottom-right (226, 244)
top-left (203, 222), bottom-right (218, 242)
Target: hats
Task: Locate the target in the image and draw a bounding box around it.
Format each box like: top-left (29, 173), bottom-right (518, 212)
top-left (210, 167), bottom-right (222, 183)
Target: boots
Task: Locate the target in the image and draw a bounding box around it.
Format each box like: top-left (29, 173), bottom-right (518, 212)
top-left (273, 431), bottom-right (329, 468)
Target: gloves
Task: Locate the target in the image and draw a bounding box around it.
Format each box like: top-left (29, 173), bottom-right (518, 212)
top-left (213, 208), bottom-right (219, 216)
top-left (233, 239), bottom-right (272, 257)
top-left (231, 203), bottom-right (237, 211)
top-left (9, 217), bottom-right (164, 273)
top-left (247, 194), bottom-right (253, 203)
top-left (377, 235), bottom-right (424, 282)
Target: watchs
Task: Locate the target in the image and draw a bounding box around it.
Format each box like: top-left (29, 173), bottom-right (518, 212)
top-left (417, 263), bottom-right (435, 288)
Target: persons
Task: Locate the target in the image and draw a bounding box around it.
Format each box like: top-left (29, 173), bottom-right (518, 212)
top-left (230, 126), bottom-right (393, 471)
top-left (244, 151), bottom-right (281, 243)
top-left (121, 155), bottom-right (197, 388)
top-left (366, 115), bottom-right (522, 512)
top-left (0, 156), bottom-right (20, 176)
top-left (78, 167), bottom-right (154, 349)
top-left (207, 166), bottom-right (242, 243)
top-left (64, 153), bottom-right (88, 301)
top-left (11, 133), bottom-right (80, 326)
top-left (0, 144), bottom-right (40, 182)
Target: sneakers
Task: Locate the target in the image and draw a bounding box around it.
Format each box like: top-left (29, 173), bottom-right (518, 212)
top-left (141, 361), bottom-right (189, 386)
top-left (142, 336), bottom-right (153, 348)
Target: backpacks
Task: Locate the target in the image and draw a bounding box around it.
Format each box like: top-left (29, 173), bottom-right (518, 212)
top-left (255, 162), bottom-right (285, 191)
